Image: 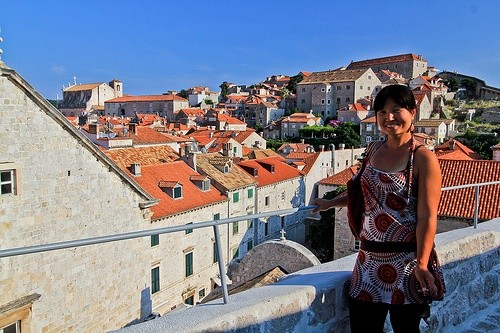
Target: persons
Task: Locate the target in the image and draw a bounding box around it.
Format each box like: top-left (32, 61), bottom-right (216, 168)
top-left (311, 84), bottom-right (446, 333)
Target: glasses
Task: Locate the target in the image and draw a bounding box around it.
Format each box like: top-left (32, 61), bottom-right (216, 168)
top-left (419, 296), bottom-right (430, 318)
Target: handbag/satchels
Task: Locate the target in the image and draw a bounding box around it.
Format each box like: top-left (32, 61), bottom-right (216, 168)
top-left (347, 141), bottom-right (381, 240)
